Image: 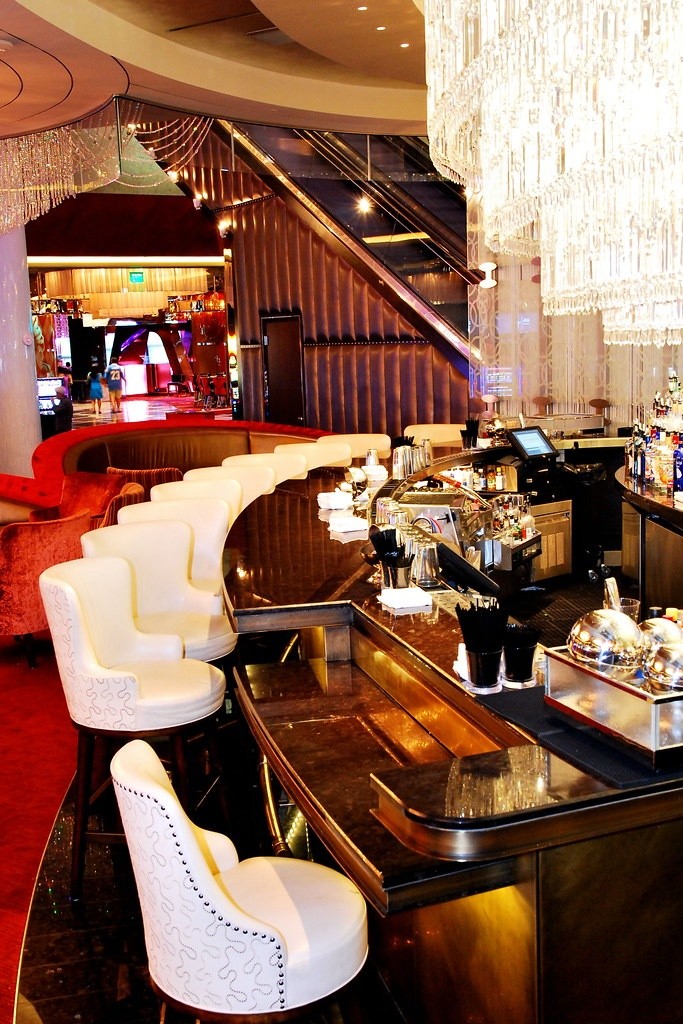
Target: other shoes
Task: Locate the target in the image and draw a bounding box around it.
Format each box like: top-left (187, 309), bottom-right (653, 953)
top-left (118, 409), bottom-right (122, 411)
top-left (99, 412), bottom-right (101, 414)
top-left (112, 409), bottom-right (117, 412)
top-left (93, 411), bottom-right (96, 413)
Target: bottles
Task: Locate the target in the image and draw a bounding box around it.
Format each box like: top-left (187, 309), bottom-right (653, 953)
top-left (440, 465), bottom-right (505, 492)
top-left (648, 606), bottom-right (682, 626)
top-left (493, 495), bottom-right (538, 561)
top-left (624, 367), bottom-right (683, 494)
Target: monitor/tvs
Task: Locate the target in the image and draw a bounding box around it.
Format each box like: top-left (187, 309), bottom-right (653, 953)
top-left (413, 525), bottom-right (500, 600)
top-left (505, 426), bottom-right (560, 461)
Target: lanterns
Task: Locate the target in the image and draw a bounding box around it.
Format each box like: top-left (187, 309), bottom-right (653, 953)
top-left (204, 293), bottom-right (223, 311)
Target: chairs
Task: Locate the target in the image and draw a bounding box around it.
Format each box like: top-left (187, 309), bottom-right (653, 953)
top-left (36, 424), bottom-right (468, 1024)
top-left (189, 374), bottom-right (228, 408)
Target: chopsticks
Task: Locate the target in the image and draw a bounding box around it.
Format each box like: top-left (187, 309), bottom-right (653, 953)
top-left (460, 418), bottom-right (479, 448)
top-left (455, 598), bottom-right (541, 687)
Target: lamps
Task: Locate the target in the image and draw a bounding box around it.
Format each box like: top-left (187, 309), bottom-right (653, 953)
top-left (426, 0), bottom-right (683, 348)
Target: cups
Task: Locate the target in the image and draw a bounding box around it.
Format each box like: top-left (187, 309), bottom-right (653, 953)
top-left (393, 439), bottom-right (433, 480)
top-left (604, 597), bottom-right (641, 628)
top-left (502, 645), bottom-right (537, 682)
top-left (462, 439), bottom-right (471, 449)
top-left (471, 435), bottom-right (477, 447)
top-left (466, 646), bottom-right (503, 689)
top-left (360, 495), bottom-right (440, 591)
top-left (365, 450), bottom-right (378, 465)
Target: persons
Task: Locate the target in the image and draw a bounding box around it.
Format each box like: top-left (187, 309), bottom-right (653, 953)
top-left (104, 357), bottom-right (126, 412)
top-left (86, 364), bottom-right (104, 413)
top-left (58, 360), bottom-right (74, 401)
